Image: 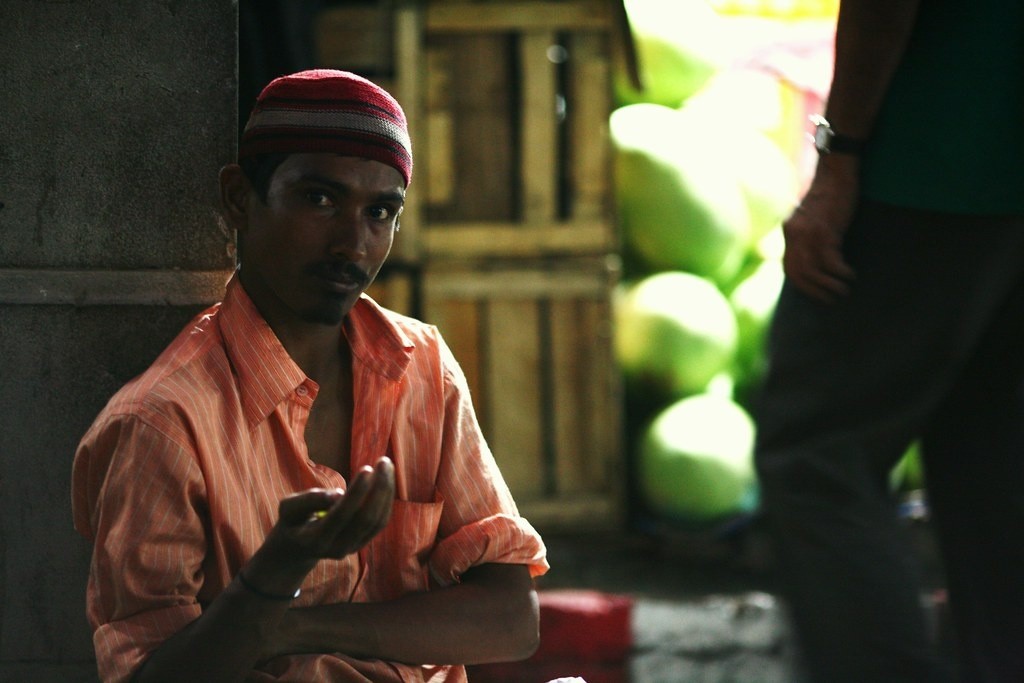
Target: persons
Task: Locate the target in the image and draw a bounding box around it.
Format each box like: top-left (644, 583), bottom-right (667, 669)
top-left (751, 0), bottom-right (1024, 683)
top-left (73, 69), bottom-right (550, 683)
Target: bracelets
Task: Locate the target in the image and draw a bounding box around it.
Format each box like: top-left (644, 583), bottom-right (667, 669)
top-left (239, 570), bottom-right (301, 599)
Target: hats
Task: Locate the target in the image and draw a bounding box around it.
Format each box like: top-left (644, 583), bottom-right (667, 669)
top-left (239, 70), bottom-right (414, 191)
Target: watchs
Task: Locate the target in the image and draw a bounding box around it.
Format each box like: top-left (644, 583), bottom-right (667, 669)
top-left (811, 115), bottom-right (867, 154)
